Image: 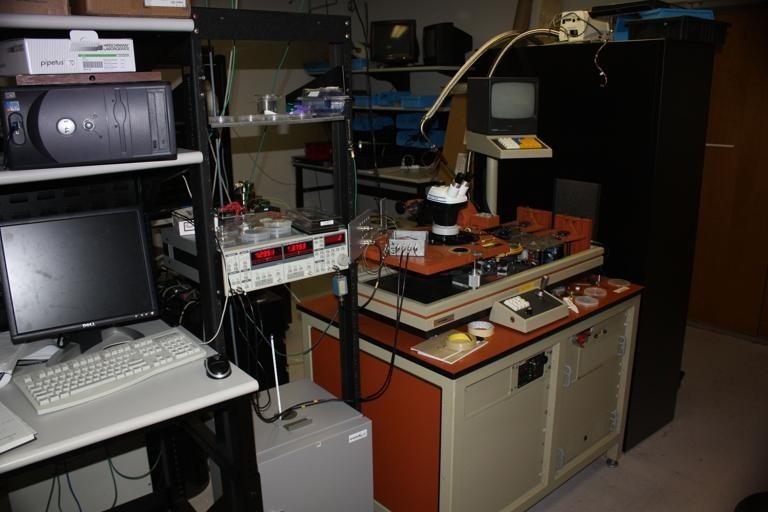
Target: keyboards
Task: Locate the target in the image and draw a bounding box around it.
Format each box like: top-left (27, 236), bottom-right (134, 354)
top-left (13, 327), bottom-right (207, 416)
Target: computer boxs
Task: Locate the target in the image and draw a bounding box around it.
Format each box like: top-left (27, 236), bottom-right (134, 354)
top-left (1, 82), bottom-right (177, 171)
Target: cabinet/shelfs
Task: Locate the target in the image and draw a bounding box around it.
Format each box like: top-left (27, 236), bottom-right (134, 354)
top-left (296, 65), bottom-right (467, 117)
top-left (1, 13), bottom-right (204, 186)
top-left (297, 290), bottom-right (642, 512)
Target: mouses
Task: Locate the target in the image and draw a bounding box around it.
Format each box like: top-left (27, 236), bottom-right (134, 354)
top-left (204, 353), bottom-right (232, 379)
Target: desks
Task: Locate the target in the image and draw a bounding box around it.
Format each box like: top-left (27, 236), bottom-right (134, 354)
top-left (1, 317), bottom-right (260, 511)
top-left (293, 156), bottom-right (436, 211)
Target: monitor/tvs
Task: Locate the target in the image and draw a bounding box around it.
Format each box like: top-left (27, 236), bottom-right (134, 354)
top-left (370, 18), bottom-right (418, 68)
top-left (0, 206), bottom-right (159, 353)
top-left (421, 21), bottom-right (473, 66)
top-left (466, 76), bottom-right (539, 135)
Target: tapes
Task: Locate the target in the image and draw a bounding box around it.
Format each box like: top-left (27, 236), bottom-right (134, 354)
top-left (467, 321), bottom-right (495, 338)
top-left (446, 332), bottom-right (476, 352)
